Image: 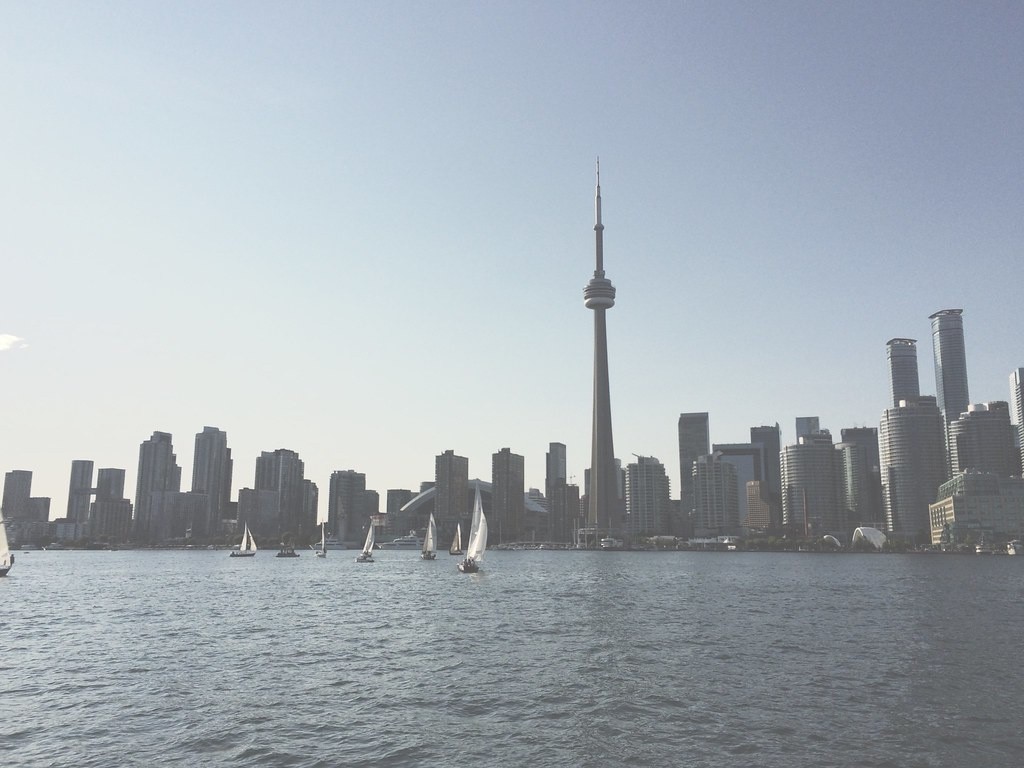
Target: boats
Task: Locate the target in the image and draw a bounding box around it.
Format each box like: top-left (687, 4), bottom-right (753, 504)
top-left (975, 535), bottom-right (992, 555)
top-left (1006, 539), bottom-right (1023, 555)
top-left (275, 542), bottom-right (300, 557)
top-left (309, 531), bottom-right (350, 550)
top-left (374, 528), bottom-right (425, 550)
top-left (991, 549), bottom-right (1007, 555)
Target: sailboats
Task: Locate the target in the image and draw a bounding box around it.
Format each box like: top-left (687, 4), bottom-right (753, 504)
top-left (229, 519), bottom-right (257, 557)
top-left (355, 520), bottom-right (375, 563)
top-left (420, 512), bottom-right (438, 560)
top-left (0, 505), bottom-right (15, 578)
top-left (315, 517), bottom-right (327, 558)
top-left (456, 478), bottom-right (488, 573)
top-left (449, 522), bottom-right (464, 555)
